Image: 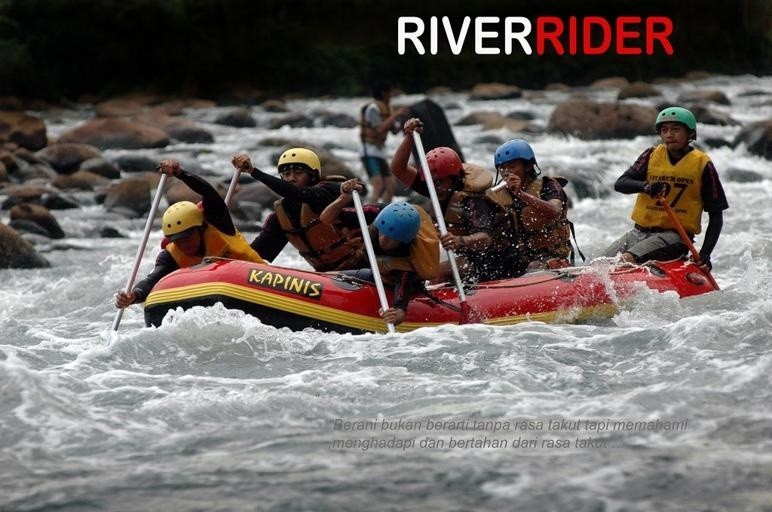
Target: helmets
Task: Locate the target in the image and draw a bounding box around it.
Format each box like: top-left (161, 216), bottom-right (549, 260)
top-left (278, 148), bottom-right (321, 175)
top-left (655, 107), bottom-right (697, 141)
top-left (420, 147), bottom-right (464, 182)
top-left (495, 139), bottom-right (536, 168)
top-left (163, 201), bottom-right (205, 235)
top-left (373, 200), bottom-right (421, 244)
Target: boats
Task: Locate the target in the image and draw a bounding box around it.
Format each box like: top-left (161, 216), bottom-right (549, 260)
top-left (143, 257), bottom-right (721, 335)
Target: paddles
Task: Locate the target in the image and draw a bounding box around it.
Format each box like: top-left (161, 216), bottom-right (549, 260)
top-left (411, 128), bottom-right (486, 324)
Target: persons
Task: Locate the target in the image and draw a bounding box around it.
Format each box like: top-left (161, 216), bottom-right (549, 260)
top-left (603, 106), bottom-right (728, 272)
top-left (114, 160), bottom-right (267, 309)
top-left (233, 147), bottom-right (351, 272)
top-left (320, 178), bottom-right (416, 324)
top-left (491, 139), bottom-right (570, 268)
top-left (359, 75), bottom-right (408, 205)
top-left (392, 118), bottom-right (500, 254)
